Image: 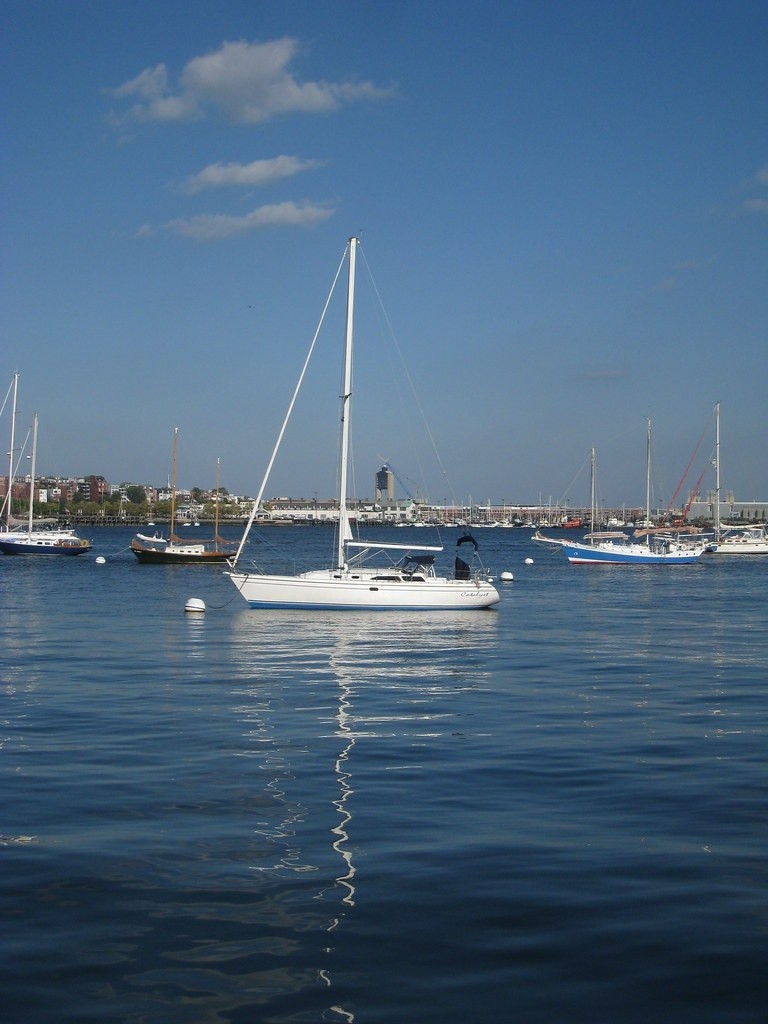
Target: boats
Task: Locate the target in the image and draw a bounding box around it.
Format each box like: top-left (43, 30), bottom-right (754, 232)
top-left (391, 518), bottom-right (684, 529)
top-left (709, 527), bottom-right (768, 554)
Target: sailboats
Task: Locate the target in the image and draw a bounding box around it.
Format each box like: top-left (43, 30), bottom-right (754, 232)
top-left (530, 416), bottom-right (707, 564)
top-left (127, 427), bottom-right (250, 564)
top-left (0, 373), bottom-right (93, 556)
top-left (221, 235), bottom-right (503, 612)
